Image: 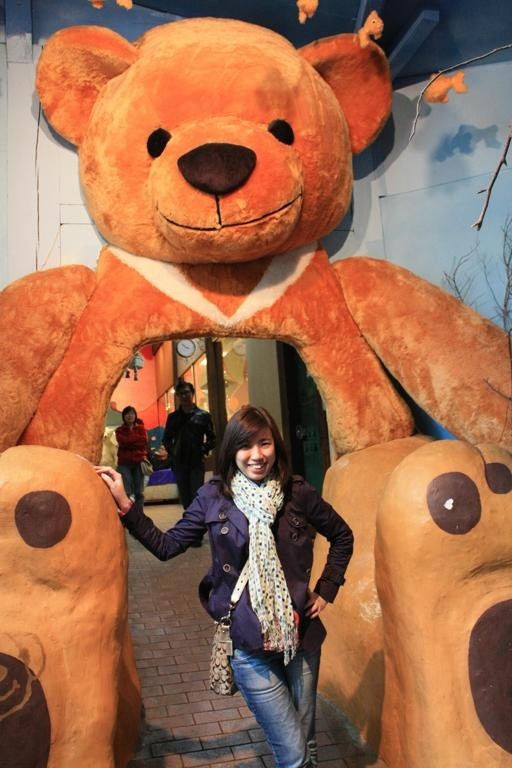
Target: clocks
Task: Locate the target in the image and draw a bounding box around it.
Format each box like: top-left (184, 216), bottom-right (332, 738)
top-left (175, 338), bottom-right (196, 358)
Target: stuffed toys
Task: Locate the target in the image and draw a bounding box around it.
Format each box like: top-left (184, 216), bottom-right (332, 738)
top-left (0, 16), bottom-right (512, 768)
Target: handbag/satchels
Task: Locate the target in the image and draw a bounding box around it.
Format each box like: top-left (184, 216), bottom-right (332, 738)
top-left (208, 616), bottom-right (239, 697)
top-left (140, 459), bottom-right (153, 474)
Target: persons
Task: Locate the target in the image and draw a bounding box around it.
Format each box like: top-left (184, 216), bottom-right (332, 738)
top-left (162, 381), bottom-right (218, 548)
top-left (94, 401), bottom-right (354, 768)
top-left (114, 406), bottom-right (150, 515)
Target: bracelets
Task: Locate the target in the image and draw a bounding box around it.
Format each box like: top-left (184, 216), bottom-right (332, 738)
top-left (121, 499), bottom-right (133, 512)
